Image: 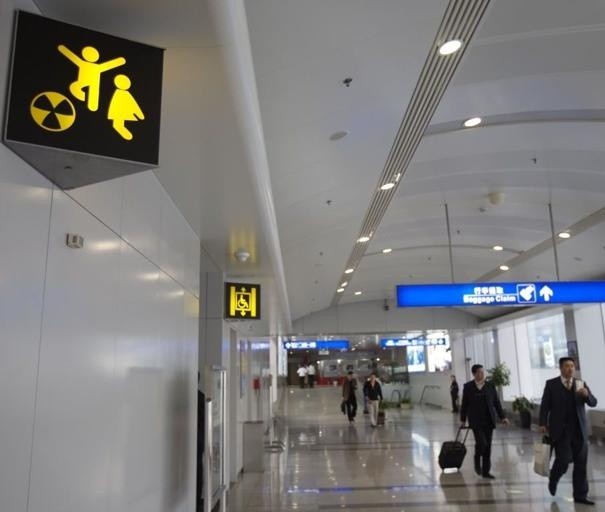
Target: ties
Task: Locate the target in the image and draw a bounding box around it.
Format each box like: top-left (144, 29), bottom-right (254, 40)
top-left (566, 380), bottom-right (572, 389)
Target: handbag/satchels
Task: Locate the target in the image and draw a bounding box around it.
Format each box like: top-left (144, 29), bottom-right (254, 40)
top-left (533, 431), bottom-right (555, 477)
top-left (340, 399), bottom-right (346, 414)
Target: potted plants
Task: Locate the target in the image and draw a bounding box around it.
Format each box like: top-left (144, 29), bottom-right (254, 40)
top-left (486, 361), bottom-right (512, 423)
top-left (511, 396), bottom-right (536, 430)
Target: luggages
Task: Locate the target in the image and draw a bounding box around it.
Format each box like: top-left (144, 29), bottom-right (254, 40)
top-left (377, 400), bottom-right (386, 426)
top-left (438, 424), bottom-right (472, 470)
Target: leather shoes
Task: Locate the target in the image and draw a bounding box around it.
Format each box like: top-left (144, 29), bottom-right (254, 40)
top-left (575, 497), bottom-right (596, 505)
top-left (548, 469), bottom-right (557, 496)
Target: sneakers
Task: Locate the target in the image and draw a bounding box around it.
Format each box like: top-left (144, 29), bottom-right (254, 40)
top-left (474, 465), bottom-right (495, 478)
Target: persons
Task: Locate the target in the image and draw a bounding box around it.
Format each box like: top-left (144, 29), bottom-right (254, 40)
top-left (343, 421), bottom-right (360, 464)
top-left (296, 361), bottom-right (316, 388)
top-left (450, 376), bottom-right (459, 412)
top-left (539, 358), bottom-right (597, 505)
top-left (342, 371), bottom-right (357, 421)
top-left (550, 501), bottom-right (589, 512)
top-left (366, 428), bottom-right (387, 476)
top-left (476, 479), bottom-right (496, 512)
top-left (460, 365), bottom-right (510, 479)
top-left (366, 374), bottom-right (383, 428)
